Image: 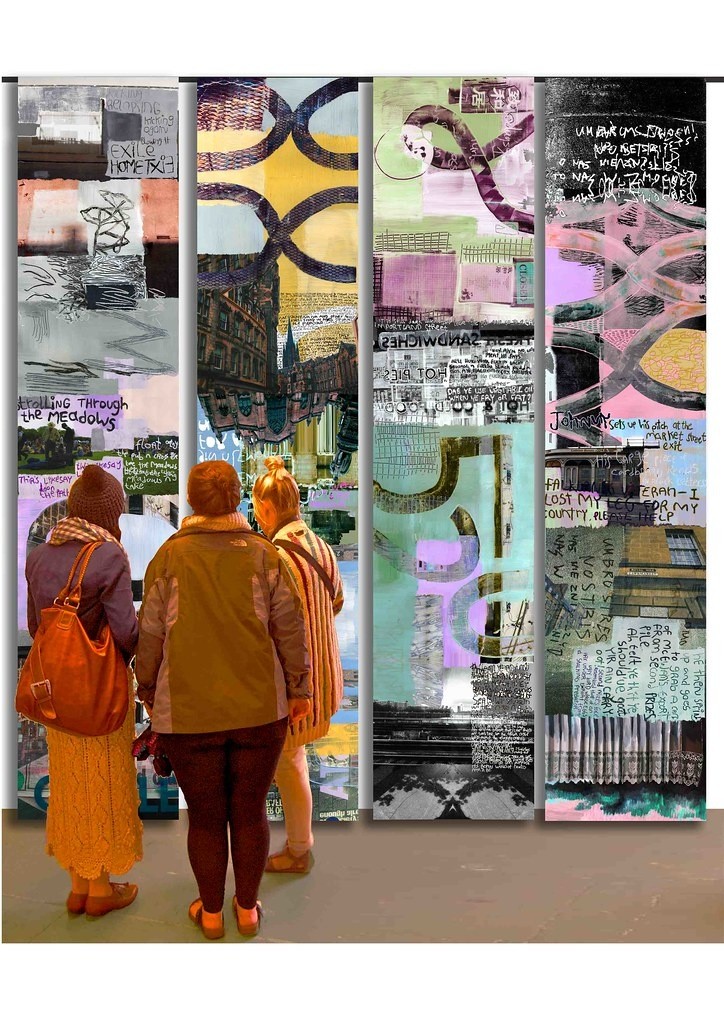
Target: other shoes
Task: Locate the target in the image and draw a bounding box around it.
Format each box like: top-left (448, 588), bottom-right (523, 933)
top-left (66, 892), bottom-right (89, 915)
top-left (85, 880), bottom-right (138, 920)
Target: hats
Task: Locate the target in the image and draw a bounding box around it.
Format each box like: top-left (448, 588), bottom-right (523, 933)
top-left (67, 464), bottom-right (125, 544)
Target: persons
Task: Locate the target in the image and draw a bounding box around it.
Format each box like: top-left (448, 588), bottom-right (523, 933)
top-left (134, 460), bottom-right (315, 938)
top-left (23, 465), bottom-right (140, 916)
top-left (252, 454), bottom-right (343, 873)
top-left (17, 423), bottom-right (93, 466)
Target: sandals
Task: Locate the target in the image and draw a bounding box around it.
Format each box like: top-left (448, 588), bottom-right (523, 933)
top-left (263, 841), bottom-right (315, 875)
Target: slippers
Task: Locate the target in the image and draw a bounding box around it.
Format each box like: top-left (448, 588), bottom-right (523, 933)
top-left (232, 894), bottom-right (262, 935)
top-left (188, 895), bottom-right (225, 939)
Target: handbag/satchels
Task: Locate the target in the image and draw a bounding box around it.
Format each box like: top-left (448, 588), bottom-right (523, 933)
top-left (15, 543), bottom-right (129, 737)
